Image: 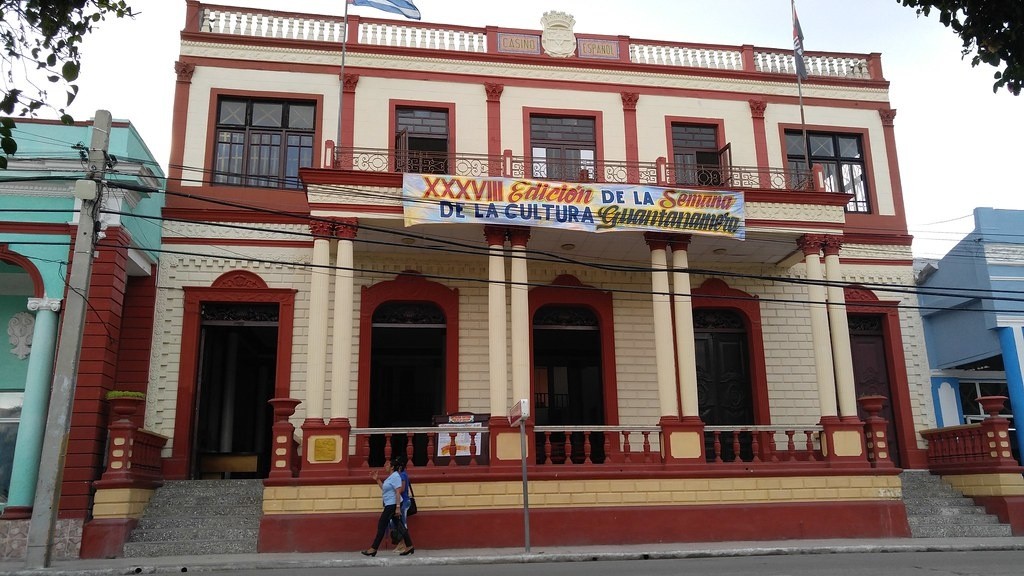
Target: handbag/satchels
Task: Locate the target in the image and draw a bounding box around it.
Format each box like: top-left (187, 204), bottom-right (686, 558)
top-left (387, 512), bottom-right (407, 546)
top-left (407, 497), bottom-right (417, 516)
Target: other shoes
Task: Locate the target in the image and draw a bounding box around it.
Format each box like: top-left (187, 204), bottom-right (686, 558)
top-left (392, 543), bottom-right (405, 552)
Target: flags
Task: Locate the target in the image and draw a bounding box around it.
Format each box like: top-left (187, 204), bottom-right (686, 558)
top-left (347, 0), bottom-right (422, 20)
top-left (792, 4), bottom-right (807, 80)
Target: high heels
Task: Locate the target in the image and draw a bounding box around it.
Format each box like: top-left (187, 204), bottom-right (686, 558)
top-left (399, 546), bottom-right (414, 555)
top-left (361, 550), bottom-right (377, 557)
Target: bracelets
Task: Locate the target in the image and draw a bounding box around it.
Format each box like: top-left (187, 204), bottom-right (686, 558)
top-left (396, 505), bottom-right (400, 507)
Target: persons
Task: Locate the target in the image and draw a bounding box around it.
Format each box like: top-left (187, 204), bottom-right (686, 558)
top-left (361, 457), bottom-right (414, 556)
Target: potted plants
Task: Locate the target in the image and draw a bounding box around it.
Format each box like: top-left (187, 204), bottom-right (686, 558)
top-left (857, 394), bottom-right (888, 420)
top-left (105, 391), bottom-right (147, 424)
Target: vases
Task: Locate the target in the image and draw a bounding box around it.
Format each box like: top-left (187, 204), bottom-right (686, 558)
top-left (974, 396), bottom-right (1009, 420)
top-left (267, 399), bottom-right (302, 423)
